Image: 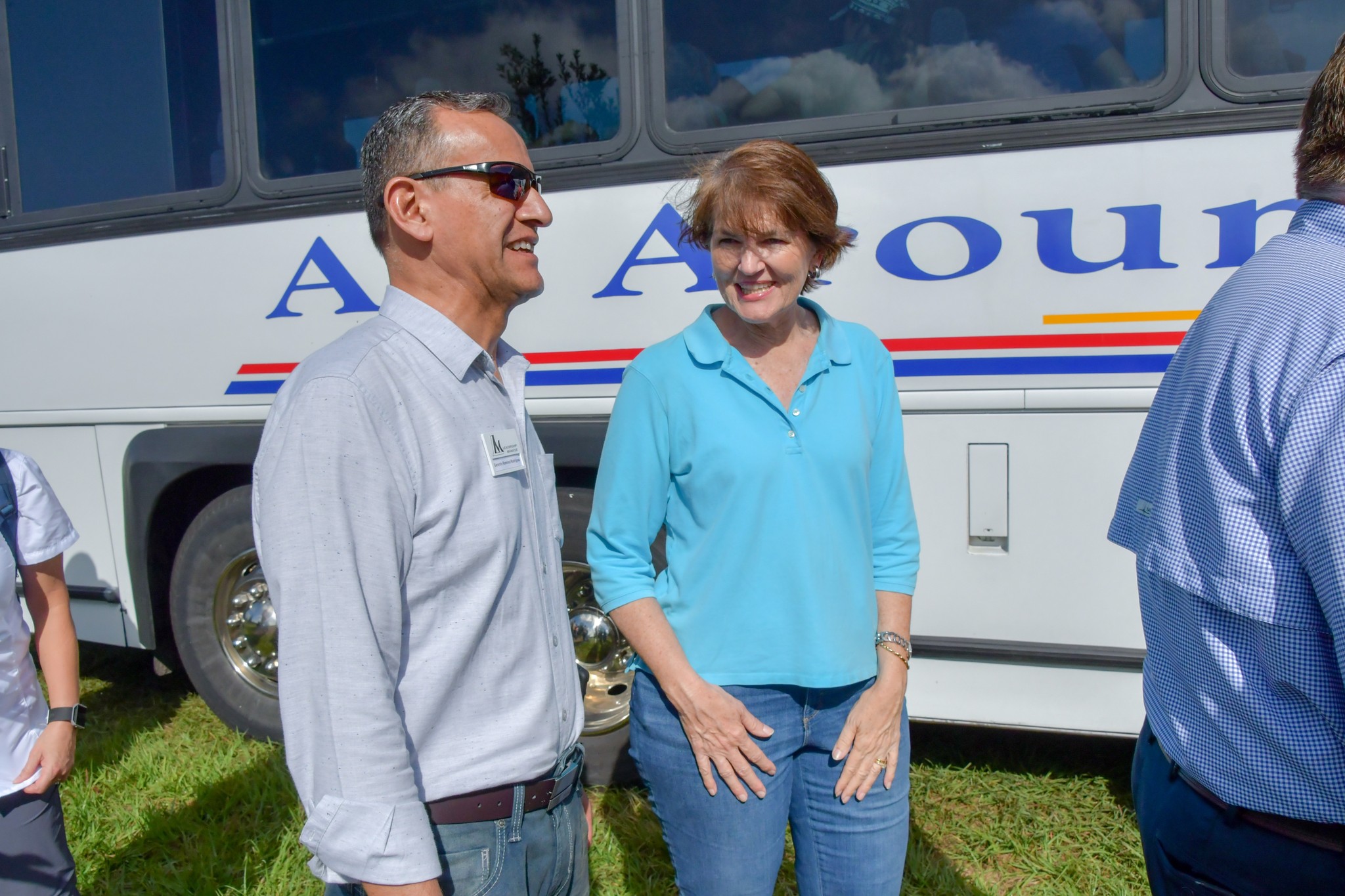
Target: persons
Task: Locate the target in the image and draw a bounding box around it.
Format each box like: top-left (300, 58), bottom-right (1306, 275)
top-left (581, 137), bottom-right (923, 893)
top-left (252, 92), bottom-right (600, 896)
top-left (1108, 34), bottom-right (1345, 896)
top-left (1, 447), bottom-right (89, 895)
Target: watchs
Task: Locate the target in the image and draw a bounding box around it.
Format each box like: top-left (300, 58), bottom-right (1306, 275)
top-left (45, 704), bottom-right (87, 731)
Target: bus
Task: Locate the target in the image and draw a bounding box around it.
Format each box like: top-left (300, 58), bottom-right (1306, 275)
top-left (0, 0), bottom-right (1345, 743)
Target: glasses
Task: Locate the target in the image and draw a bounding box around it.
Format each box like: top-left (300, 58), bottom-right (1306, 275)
top-left (376, 160), bottom-right (542, 216)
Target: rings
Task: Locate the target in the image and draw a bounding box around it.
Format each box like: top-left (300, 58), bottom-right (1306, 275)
top-left (876, 758), bottom-right (887, 767)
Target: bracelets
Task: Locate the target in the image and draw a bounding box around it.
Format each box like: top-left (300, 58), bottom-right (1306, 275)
top-left (878, 643), bottom-right (910, 668)
top-left (874, 632), bottom-right (914, 661)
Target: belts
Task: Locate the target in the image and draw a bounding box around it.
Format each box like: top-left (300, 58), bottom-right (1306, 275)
top-left (1155, 729), bottom-right (1345, 853)
top-left (424, 778), bottom-right (556, 824)
